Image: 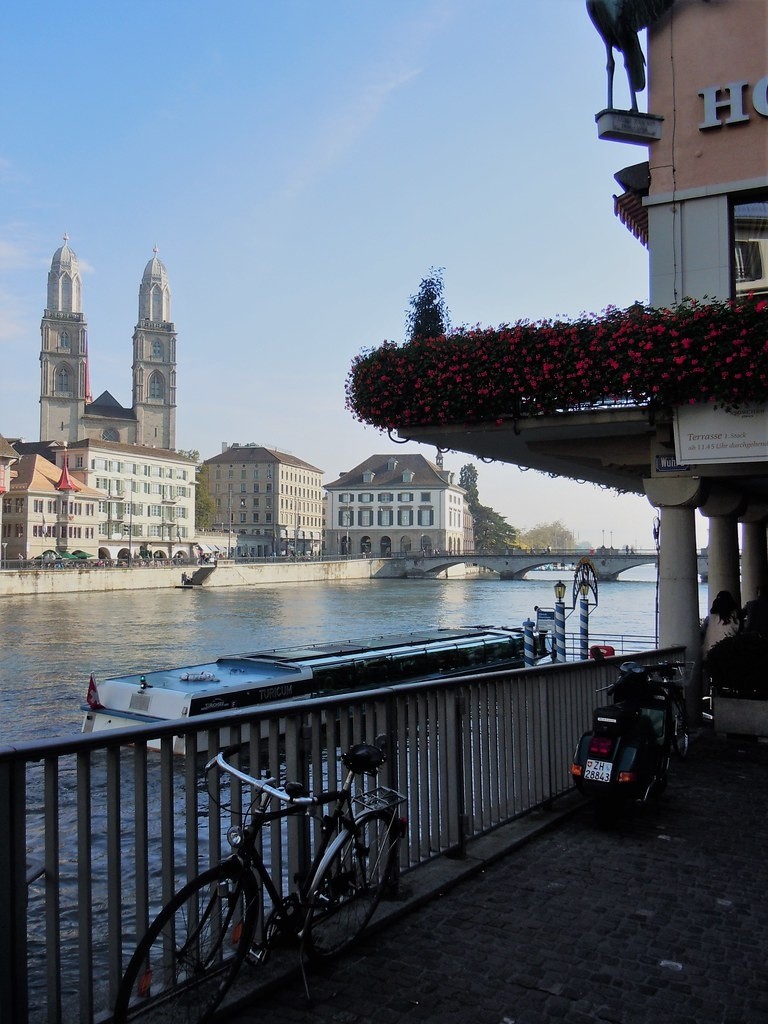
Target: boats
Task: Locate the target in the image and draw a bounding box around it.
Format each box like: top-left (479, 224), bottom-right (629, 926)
top-left (78, 618), bottom-right (553, 759)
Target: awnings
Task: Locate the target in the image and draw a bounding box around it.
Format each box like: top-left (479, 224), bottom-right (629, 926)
top-left (197, 543), bottom-right (229, 554)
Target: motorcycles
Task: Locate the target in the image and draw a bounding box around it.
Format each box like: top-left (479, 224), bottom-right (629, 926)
top-left (571, 659), bottom-right (696, 834)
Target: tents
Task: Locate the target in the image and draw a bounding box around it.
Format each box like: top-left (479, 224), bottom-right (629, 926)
top-left (35, 550), bottom-right (62, 560)
top-left (59, 551), bottom-right (78, 559)
top-left (77, 551), bottom-right (95, 559)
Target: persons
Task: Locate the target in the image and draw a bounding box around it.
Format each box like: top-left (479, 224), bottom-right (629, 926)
top-left (625, 545), bottom-right (635, 554)
top-left (17, 553), bottom-right (24, 571)
top-left (696, 590), bottom-right (741, 699)
top-left (138, 553), bottom-right (144, 567)
top-left (104, 556), bottom-right (109, 566)
top-left (202, 553), bottom-right (226, 559)
top-left (422, 546), bottom-right (440, 557)
top-left (546, 544), bottom-right (552, 554)
top-left (741, 584), bottom-right (767, 638)
top-left (181, 570), bottom-right (192, 584)
top-left (480, 544), bottom-right (489, 555)
top-left (44, 552), bottom-right (61, 569)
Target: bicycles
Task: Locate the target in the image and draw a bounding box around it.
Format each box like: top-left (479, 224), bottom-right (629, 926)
top-left (651, 660), bottom-right (692, 760)
top-left (112, 742), bottom-right (408, 1024)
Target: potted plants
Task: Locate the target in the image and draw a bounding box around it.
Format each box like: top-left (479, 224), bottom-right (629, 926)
top-left (701, 635), bottom-right (767, 743)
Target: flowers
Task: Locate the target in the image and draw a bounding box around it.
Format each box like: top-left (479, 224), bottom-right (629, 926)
top-left (343, 288), bottom-right (768, 432)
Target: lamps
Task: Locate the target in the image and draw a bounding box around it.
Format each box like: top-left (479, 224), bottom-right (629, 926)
top-left (579, 576), bottom-right (589, 595)
top-left (554, 580), bottom-right (566, 599)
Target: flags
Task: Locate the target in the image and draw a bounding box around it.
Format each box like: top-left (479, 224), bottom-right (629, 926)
top-left (85, 674), bottom-right (104, 712)
